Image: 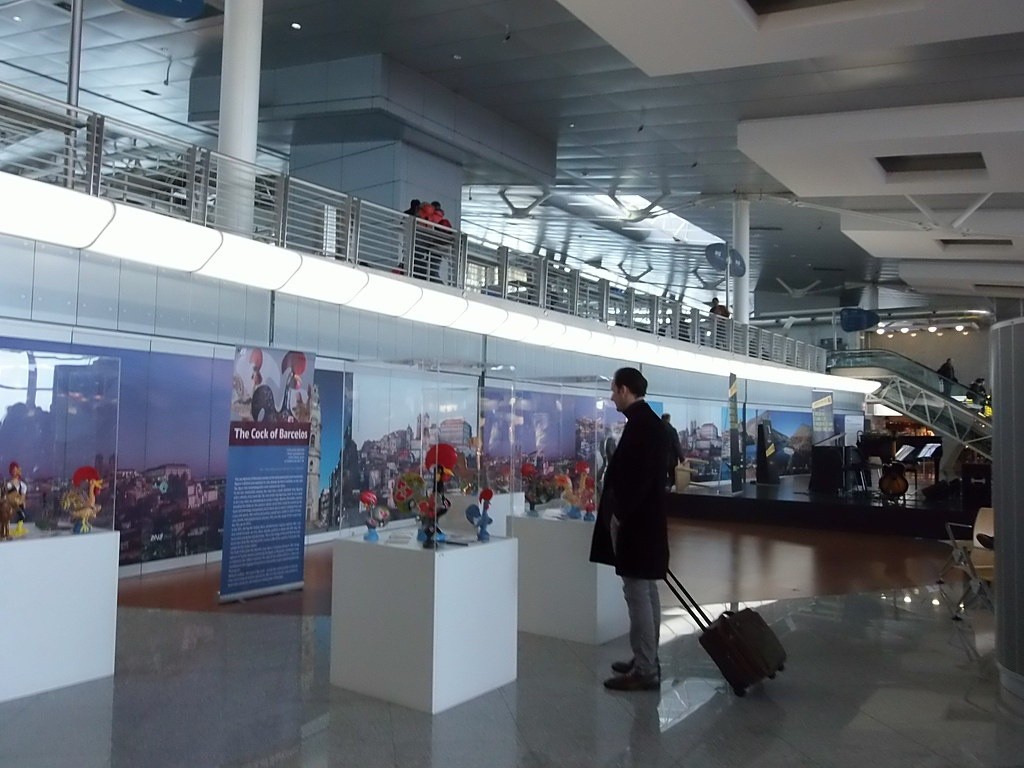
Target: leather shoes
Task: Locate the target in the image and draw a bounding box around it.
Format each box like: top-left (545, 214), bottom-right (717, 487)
top-left (612, 657), bottom-right (661, 677)
top-left (603, 671), bottom-right (661, 691)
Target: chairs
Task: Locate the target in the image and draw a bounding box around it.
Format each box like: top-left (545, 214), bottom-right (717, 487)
top-left (938, 507), bottom-right (994, 619)
top-left (675, 455), bottom-right (710, 493)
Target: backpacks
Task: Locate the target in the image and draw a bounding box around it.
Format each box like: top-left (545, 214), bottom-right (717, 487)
top-left (966, 384), bottom-right (976, 399)
top-left (435, 218), bottom-right (454, 245)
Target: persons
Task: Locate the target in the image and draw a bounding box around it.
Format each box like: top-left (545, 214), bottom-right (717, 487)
top-left (589, 368), bottom-right (675, 691)
top-left (938, 358), bottom-right (958, 397)
top-left (660, 413), bottom-right (685, 492)
top-left (972, 378), bottom-right (986, 415)
top-left (400, 199), bottom-right (445, 284)
top-left (709, 298), bottom-right (730, 320)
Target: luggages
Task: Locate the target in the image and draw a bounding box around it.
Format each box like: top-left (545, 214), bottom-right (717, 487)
top-left (664, 568), bottom-right (787, 697)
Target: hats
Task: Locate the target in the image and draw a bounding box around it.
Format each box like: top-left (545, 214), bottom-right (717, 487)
top-left (976, 378), bottom-right (982, 383)
top-left (712, 298), bottom-right (718, 303)
